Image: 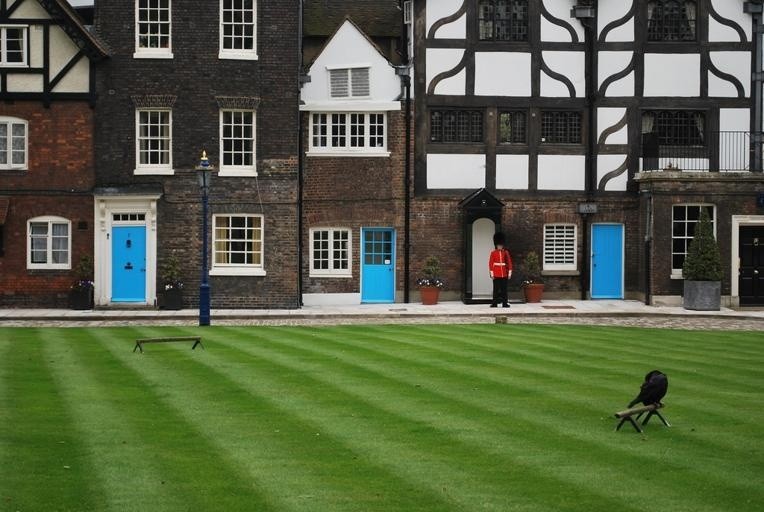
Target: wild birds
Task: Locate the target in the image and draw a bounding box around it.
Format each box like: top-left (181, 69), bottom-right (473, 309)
top-left (626, 370), bottom-right (668, 410)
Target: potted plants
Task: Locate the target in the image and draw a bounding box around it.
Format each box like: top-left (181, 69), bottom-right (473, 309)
top-left (680, 206), bottom-right (725, 310)
top-left (159, 256), bottom-right (189, 309)
top-left (416, 254), bottom-right (443, 304)
top-left (523, 250), bottom-right (544, 303)
top-left (70, 252), bottom-right (93, 308)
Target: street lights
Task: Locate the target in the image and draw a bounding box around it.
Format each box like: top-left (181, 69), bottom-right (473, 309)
top-left (191, 151), bottom-right (214, 325)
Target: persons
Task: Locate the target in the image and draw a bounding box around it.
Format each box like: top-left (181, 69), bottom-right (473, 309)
top-left (489, 232), bottom-right (513, 307)
